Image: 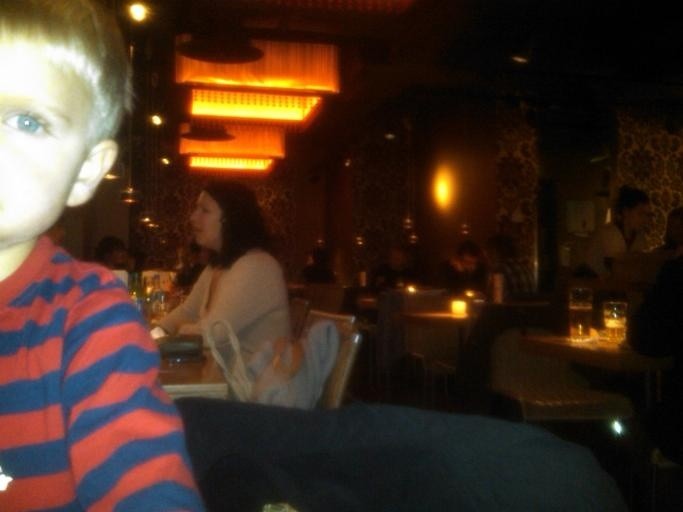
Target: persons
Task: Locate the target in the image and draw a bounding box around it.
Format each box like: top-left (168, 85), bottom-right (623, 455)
top-left (151, 182), bottom-right (311, 404)
top-left (0, 0), bottom-right (200, 511)
top-left (171, 243), bottom-right (202, 289)
top-left (630, 207), bottom-right (682, 466)
top-left (305, 250), bottom-right (330, 282)
top-left (439, 239), bottom-right (488, 286)
top-left (93, 234), bottom-right (127, 269)
top-left (567, 188), bottom-right (652, 297)
top-left (374, 240), bottom-right (424, 289)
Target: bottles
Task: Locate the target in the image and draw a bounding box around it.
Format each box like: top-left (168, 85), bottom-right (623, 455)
top-left (156, 335), bottom-right (204, 359)
top-left (131, 272), bottom-right (175, 328)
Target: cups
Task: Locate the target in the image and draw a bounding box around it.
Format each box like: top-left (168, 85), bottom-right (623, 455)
top-left (602, 301), bottom-right (628, 343)
top-left (451, 300), bottom-right (465, 314)
top-left (568, 289), bottom-right (594, 341)
